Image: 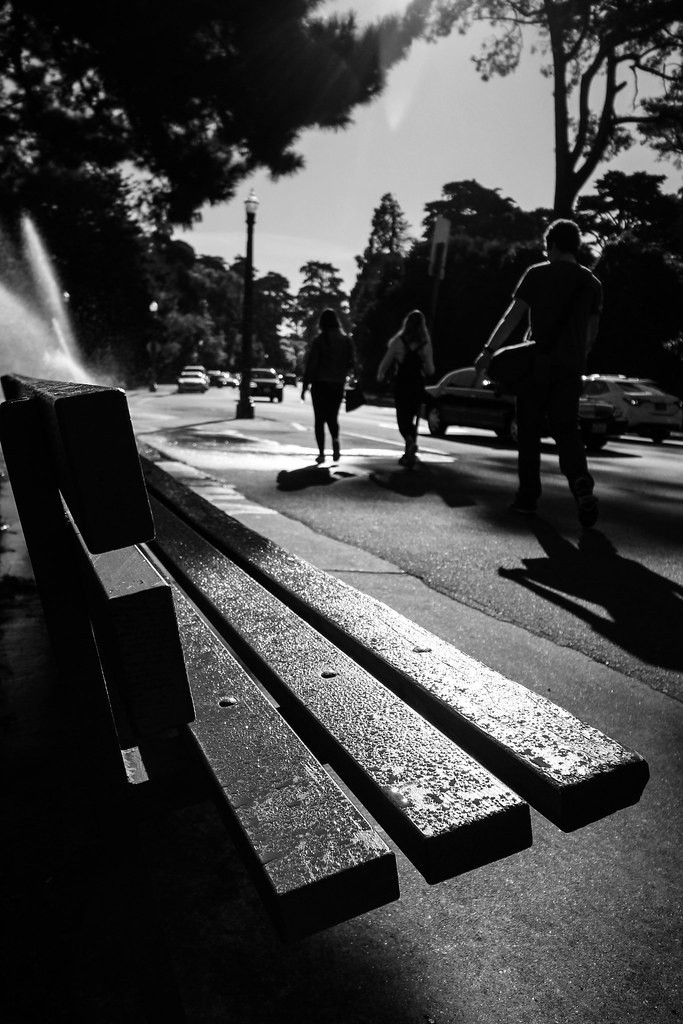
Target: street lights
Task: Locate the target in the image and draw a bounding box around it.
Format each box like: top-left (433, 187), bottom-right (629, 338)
top-left (234, 187), bottom-right (261, 418)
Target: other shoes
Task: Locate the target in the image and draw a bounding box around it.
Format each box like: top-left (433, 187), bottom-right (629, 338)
top-left (397, 442), bottom-right (421, 465)
top-left (570, 473), bottom-right (600, 530)
top-left (316, 455), bottom-right (325, 462)
top-left (332, 439), bottom-right (340, 462)
top-left (509, 498), bottom-right (538, 514)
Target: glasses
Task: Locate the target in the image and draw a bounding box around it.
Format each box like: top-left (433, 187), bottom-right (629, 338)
top-left (542, 249), bottom-right (548, 257)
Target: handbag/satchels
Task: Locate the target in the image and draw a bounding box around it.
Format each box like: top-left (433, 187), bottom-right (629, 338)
top-left (346, 384), bottom-right (367, 411)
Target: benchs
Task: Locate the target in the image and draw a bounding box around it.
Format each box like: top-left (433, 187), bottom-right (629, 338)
top-left (0, 370), bottom-right (653, 1024)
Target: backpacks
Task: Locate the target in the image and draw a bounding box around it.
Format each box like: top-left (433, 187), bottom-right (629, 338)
top-left (394, 336), bottom-right (428, 391)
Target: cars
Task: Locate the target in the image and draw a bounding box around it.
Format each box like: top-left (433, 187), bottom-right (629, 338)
top-left (175, 364), bottom-right (242, 392)
top-left (420, 366), bottom-right (614, 455)
top-left (284, 374), bottom-right (298, 387)
top-left (579, 373), bottom-right (683, 444)
top-left (249, 368), bottom-right (284, 404)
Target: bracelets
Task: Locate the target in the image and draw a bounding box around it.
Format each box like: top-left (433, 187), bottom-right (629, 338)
top-left (484, 344), bottom-right (494, 353)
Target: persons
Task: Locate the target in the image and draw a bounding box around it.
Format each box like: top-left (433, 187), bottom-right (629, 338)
top-left (377, 310), bottom-right (435, 464)
top-left (301, 308), bottom-right (356, 463)
top-left (474, 218), bottom-right (603, 527)
